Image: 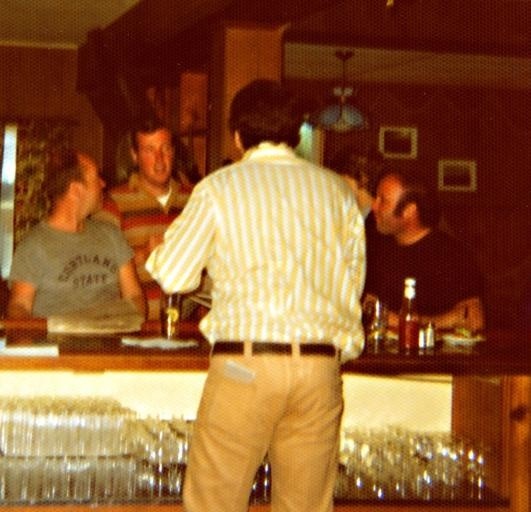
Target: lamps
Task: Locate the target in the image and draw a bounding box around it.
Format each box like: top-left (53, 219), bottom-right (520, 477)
top-left (303, 45), bottom-right (372, 137)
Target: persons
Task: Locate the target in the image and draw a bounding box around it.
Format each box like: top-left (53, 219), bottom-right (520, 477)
top-left (359, 165), bottom-right (487, 335)
top-left (326, 133), bottom-right (386, 228)
top-left (144, 77), bottom-right (368, 512)
top-left (6, 147), bottom-right (150, 326)
top-left (85, 114), bottom-right (203, 321)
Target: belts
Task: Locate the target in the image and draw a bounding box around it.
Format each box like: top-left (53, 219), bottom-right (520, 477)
top-left (211, 340), bottom-right (343, 361)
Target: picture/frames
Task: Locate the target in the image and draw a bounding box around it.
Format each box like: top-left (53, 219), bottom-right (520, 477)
top-left (378, 124), bottom-right (418, 160)
top-left (436, 157), bottom-right (479, 195)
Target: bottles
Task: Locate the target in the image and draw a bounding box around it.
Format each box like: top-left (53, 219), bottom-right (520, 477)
top-left (399, 278), bottom-right (420, 355)
top-left (161, 290), bottom-right (183, 338)
